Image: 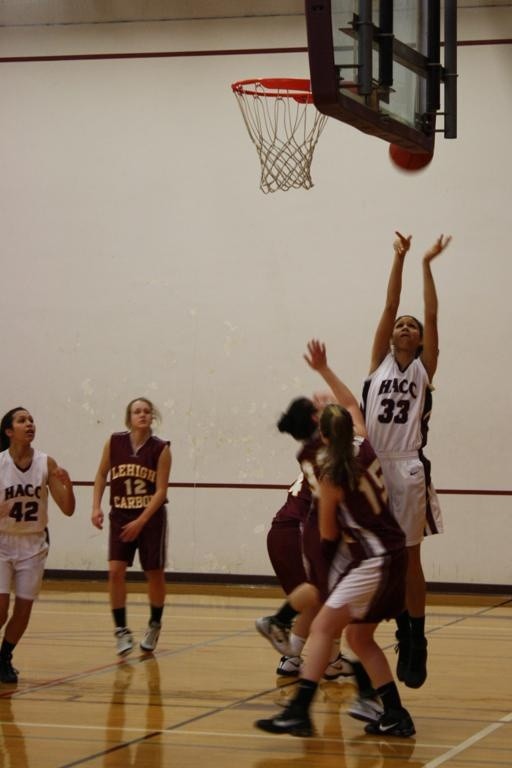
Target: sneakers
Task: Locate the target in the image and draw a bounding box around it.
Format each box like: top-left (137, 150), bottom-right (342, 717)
top-left (254, 614), bottom-right (296, 659)
top-left (139, 653), bottom-right (163, 687)
top-left (275, 679), bottom-right (364, 708)
top-left (140, 620), bottom-right (162, 652)
top-left (255, 712), bottom-right (316, 739)
top-left (363, 707), bottom-right (417, 739)
top-left (0, 653), bottom-right (18, 683)
top-left (112, 662), bottom-right (136, 694)
top-left (392, 628), bottom-right (430, 690)
top-left (320, 652), bottom-right (357, 681)
top-left (346, 694), bottom-right (386, 724)
top-left (276, 653), bottom-right (302, 678)
top-left (374, 736), bottom-right (417, 760)
top-left (113, 625), bottom-right (139, 658)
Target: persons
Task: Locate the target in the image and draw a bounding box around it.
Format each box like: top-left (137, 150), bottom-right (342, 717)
top-left (1, 407), bottom-right (76, 685)
top-left (103, 654), bottom-right (164, 767)
top-left (358, 229), bottom-right (453, 689)
top-left (91, 396), bottom-right (172, 659)
top-left (259, 676), bottom-right (426, 768)
top-left (0, 686), bottom-right (29, 768)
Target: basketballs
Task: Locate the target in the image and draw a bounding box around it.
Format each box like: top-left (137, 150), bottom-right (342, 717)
top-left (389, 143), bottom-right (433, 170)
